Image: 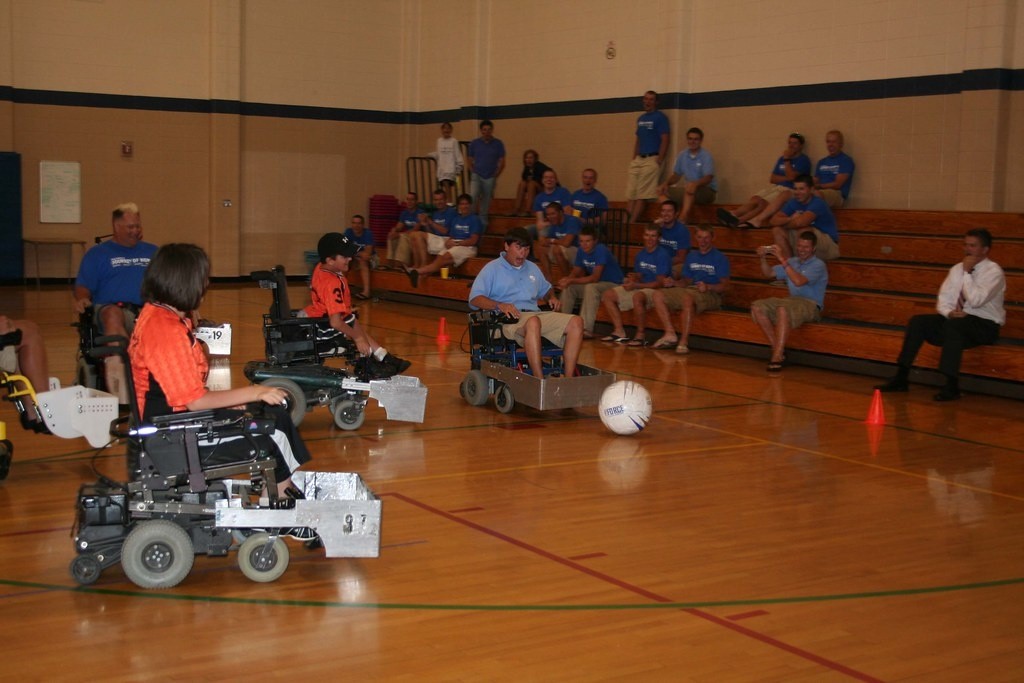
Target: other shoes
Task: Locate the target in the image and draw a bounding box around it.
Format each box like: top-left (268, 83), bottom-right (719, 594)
top-left (410, 270), bottom-right (418, 287)
top-left (935, 392), bottom-right (959, 400)
top-left (279, 526), bottom-right (318, 540)
top-left (716, 207), bottom-right (739, 228)
top-left (873, 384), bottom-right (909, 392)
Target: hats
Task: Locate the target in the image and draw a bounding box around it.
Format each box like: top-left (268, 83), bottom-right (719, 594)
top-left (317, 231), bottom-right (360, 257)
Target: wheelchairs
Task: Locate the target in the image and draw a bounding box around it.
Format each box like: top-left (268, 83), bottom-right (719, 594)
top-left (0, 328), bottom-right (119, 484)
top-left (66, 334), bottom-right (383, 592)
top-left (241, 266), bottom-right (429, 431)
top-left (459, 307), bottom-right (617, 414)
top-left (69, 233), bottom-right (233, 393)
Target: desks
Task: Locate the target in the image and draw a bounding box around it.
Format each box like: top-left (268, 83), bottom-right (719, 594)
top-left (23, 237), bottom-right (87, 293)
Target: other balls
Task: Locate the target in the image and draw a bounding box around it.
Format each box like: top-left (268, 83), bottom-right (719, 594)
top-left (598, 380), bottom-right (653, 435)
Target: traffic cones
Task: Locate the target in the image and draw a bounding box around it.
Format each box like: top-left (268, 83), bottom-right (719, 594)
top-left (435, 319), bottom-right (450, 342)
top-left (859, 388), bottom-right (887, 425)
top-left (866, 423), bottom-right (885, 459)
top-left (437, 342), bottom-right (450, 370)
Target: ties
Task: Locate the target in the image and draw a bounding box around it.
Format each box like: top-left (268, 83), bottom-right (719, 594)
top-left (955, 292), bottom-right (966, 312)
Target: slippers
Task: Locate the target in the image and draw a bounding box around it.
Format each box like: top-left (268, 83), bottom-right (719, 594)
top-left (356, 292), bottom-right (371, 299)
top-left (601, 334), bottom-right (629, 342)
top-left (675, 345), bottom-right (689, 354)
top-left (629, 338), bottom-right (647, 346)
top-left (650, 339), bottom-right (672, 349)
top-left (737, 221), bottom-right (759, 229)
top-left (766, 361), bottom-right (782, 371)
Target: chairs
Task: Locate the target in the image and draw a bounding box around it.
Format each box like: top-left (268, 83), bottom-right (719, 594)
top-left (270, 265), bottom-right (344, 359)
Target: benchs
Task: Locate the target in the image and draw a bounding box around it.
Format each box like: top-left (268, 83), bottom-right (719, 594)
top-left (348, 199), bottom-right (1024, 399)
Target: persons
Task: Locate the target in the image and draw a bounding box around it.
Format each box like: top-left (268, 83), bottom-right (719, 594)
top-left (344, 215), bottom-right (379, 300)
top-left (540, 202), bottom-right (587, 280)
top-left (73, 202), bottom-right (160, 412)
top-left (127, 243), bottom-right (320, 540)
top-left (0, 315), bottom-right (50, 427)
top-left (654, 200), bottom-right (691, 275)
top-left (649, 226), bottom-right (730, 354)
top-left (374, 121), bottom-right (573, 288)
top-left (751, 231), bottom-right (828, 371)
top-left (736, 131), bottom-right (854, 229)
top-left (563, 169), bottom-right (608, 233)
top-left (622, 91), bottom-right (670, 224)
top-left (601, 223), bottom-right (672, 345)
top-left (873, 227), bottom-right (1006, 398)
top-left (468, 226), bottom-right (583, 379)
top-left (558, 225), bottom-right (624, 339)
top-left (297, 232), bottom-right (411, 379)
top-left (770, 173), bottom-right (840, 266)
top-left (658, 127), bottom-right (716, 225)
top-left (717, 132), bottom-right (810, 226)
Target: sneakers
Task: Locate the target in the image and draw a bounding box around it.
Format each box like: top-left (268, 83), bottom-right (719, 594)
top-left (381, 351), bottom-right (411, 373)
top-left (354, 356), bottom-right (396, 378)
